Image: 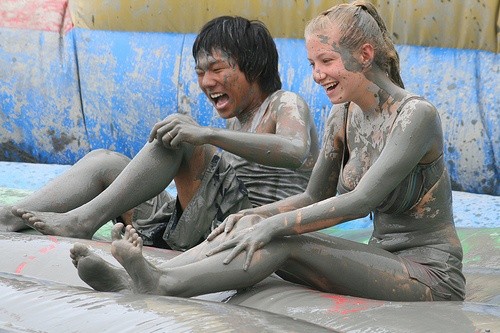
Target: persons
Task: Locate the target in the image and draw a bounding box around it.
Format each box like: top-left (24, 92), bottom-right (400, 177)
top-left (0, 16), bottom-right (320, 251)
top-left (70, 1), bottom-right (466, 304)
